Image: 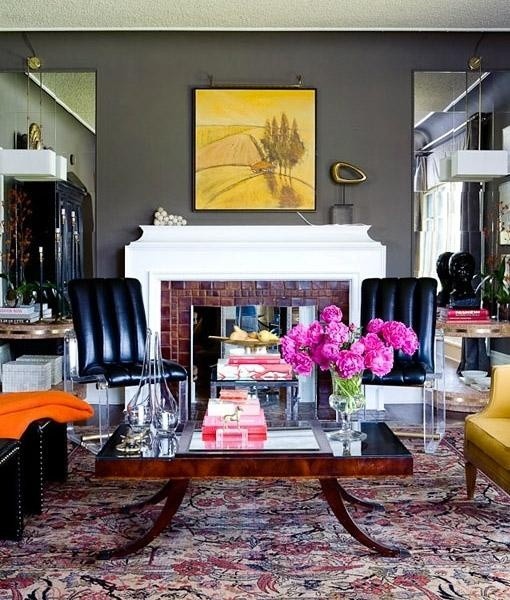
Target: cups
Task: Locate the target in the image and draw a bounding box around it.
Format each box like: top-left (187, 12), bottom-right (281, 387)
top-left (120, 434), bottom-right (137, 447)
top-left (151, 412), bottom-right (180, 436)
top-left (129, 405), bottom-right (152, 433)
top-left (151, 430), bottom-right (179, 458)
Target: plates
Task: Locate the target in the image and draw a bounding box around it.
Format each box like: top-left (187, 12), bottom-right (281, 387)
top-left (116, 443), bottom-right (141, 454)
top-left (471, 384), bottom-right (490, 393)
top-left (458, 376), bottom-right (474, 384)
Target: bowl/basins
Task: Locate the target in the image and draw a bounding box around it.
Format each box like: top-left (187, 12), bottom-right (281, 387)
top-left (473, 377), bottom-right (491, 387)
top-left (460, 370), bottom-right (488, 380)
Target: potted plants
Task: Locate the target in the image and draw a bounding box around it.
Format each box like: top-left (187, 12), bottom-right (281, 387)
top-left (475, 255), bottom-right (510, 322)
top-left (472, 256), bottom-right (500, 316)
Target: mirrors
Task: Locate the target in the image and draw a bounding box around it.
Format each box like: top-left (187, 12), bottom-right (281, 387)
top-left (409, 68), bottom-right (509, 318)
top-left (0, 69), bottom-right (97, 326)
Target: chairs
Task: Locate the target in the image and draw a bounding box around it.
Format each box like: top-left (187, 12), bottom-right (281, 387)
top-left (347, 277), bottom-right (446, 455)
top-left (0, 393), bottom-right (67, 541)
top-left (68, 277), bottom-right (187, 432)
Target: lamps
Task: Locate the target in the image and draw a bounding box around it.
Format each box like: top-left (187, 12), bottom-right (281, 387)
top-left (439, 60), bottom-right (468, 183)
top-left (15, 59), bottom-right (69, 182)
top-left (451, 54), bottom-right (510, 182)
top-left (0, 55), bottom-right (55, 177)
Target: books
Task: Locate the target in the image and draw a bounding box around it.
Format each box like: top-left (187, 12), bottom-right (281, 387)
top-left (437, 308), bottom-right (492, 323)
top-left (0, 305), bottom-right (40, 325)
top-left (19, 303), bottom-right (52, 319)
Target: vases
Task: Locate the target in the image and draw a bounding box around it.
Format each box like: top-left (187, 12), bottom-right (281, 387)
top-left (324, 369), bottom-right (368, 442)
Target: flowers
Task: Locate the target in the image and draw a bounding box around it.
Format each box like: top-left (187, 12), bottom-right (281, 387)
top-left (281, 304), bottom-right (419, 415)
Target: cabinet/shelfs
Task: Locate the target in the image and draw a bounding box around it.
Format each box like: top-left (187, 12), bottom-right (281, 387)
top-left (10, 177), bottom-right (85, 319)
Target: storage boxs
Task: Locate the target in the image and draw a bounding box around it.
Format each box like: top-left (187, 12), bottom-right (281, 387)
top-left (2, 359), bottom-right (52, 393)
top-left (16, 354), bottom-right (63, 386)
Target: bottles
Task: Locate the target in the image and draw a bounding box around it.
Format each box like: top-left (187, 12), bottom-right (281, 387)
top-left (126, 327), bottom-right (153, 412)
top-left (150, 330), bottom-right (180, 412)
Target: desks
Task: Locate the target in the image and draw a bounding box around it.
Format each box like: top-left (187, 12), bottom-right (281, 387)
top-left (433, 322), bottom-right (510, 414)
top-left (0, 321), bottom-right (74, 339)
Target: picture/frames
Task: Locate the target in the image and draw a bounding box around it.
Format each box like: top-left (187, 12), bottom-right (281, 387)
top-left (191, 86), bottom-right (317, 213)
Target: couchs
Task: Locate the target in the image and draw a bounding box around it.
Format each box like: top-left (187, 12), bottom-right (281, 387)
top-left (464, 363), bottom-right (510, 499)
